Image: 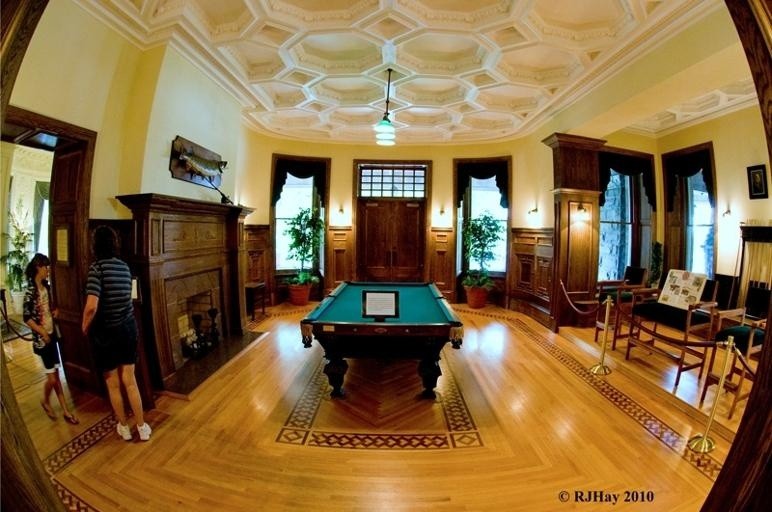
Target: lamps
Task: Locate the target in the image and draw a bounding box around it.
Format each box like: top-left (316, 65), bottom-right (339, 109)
top-left (372, 69), bottom-right (398, 146)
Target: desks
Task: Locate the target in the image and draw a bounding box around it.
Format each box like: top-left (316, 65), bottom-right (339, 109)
top-left (300, 280), bottom-right (466, 402)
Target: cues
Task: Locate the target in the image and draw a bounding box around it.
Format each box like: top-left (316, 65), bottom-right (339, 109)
top-left (736, 218), bottom-right (772, 307)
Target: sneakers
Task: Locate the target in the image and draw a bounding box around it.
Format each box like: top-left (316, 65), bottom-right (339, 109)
top-left (117, 422), bottom-right (132, 441)
top-left (40, 401), bottom-right (58, 423)
top-left (62, 413), bottom-right (80, 425)
top-left (137, 423), bottom-right (153, 440)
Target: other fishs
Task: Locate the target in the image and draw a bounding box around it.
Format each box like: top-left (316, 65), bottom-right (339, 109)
top-left (179, 143), bottom-right (228, 177)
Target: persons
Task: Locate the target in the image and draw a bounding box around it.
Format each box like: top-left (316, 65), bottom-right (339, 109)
top-left (22, 253), bottom-right (79, 424)
top-left (82, 226), bottom-right (152, 441)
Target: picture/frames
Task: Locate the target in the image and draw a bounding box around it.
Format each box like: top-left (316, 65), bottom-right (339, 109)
top-left (746, 164), bottom-right (769, 200)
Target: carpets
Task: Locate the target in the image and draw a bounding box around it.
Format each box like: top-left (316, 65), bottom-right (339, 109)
top-left (0, 317), bottom-right (33, 343)
top-left (247, 312), bottom-right (272, 330)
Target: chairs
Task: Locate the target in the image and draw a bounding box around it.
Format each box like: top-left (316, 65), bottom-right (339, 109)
top-left (596, 264), bottom-right (767, 419)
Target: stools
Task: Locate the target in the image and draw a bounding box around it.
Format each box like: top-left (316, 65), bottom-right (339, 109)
top-left (244, 281), bottom-right (269, 320)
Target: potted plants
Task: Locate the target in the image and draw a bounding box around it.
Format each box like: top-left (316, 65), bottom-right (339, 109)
top-left (459, 212), bottom-right (509, 309)
top-left (279, 203), bottom-right (327, 307)
top-left (1, 184), bottom-right (42, 317)
